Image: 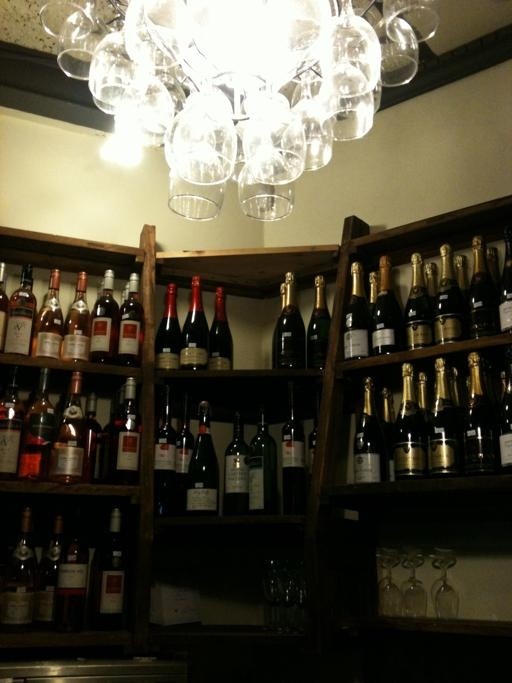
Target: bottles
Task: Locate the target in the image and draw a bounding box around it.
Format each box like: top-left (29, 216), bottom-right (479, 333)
top-left (404, 252), bottom-right (434, 351)
top-left (309, 390), bottom-right (321, 488)
top-left (394, 361), bottom-right (427, 479)
top-left (353, 376), bottom-right (387, 485)
top-left (0, 262), bottom-right (11, 354)
top-left (154, 383), bottom-right (177, 518)
top-left (496, 224), bottom-right (512, 336)
top-left (83, 391), bottom-right (103, 484)
top-left (281, 380), bottom-right (307, 514)
top-left (124, 281), bottom-right (130, 302)
top-left (174, 393), bottom-right (195, 516)
top-left (222, 409), bottom-right (248, 516)
top-left (306, 274), bottom-right (332, 369)
top-left (275, 270), bottom-right (307, 369)
top-left (248, 406), bottom-right (280, 515)
top-left (369, 254), bottom-right (407, 355)
top-left (102, 401), bottom-right (116, 484)
top-left (118, 385), bottom-right (125, 413)
top-left (427, 357), bottom-right (463, 478)
top-left (272, 283), bottom-right (286, 370)
top-left (0, 506), bottom-right (39, 634)
top-left (155, 282), bottom-right (181, 370)
top-left (0, 365), bottom-right (25, 481)
top-left (455, 254), bottom-right (471, 302)
top-left (17, 367), bottom-right (56, 483)
top-left (89, 268), bottom-right (121, 365)
top-left (495, 348), bottom-right (512, 476)
top-left (87, 505), bottom-right (130, 630)
top-left (30, 273), bottom-right (53, 358)
top-left (466, 234), bottom-right (499, 339)
top-left (117, 272), bottom-right (144, 367)
top-left (424, 263), bottom-right (439, 309)
top-left (32, 510), bottom-right (65, 632)
top-left (342, 261), bottom-right (371, 360)
top-left (378, 386), bottom-right (396, 483)
top-left (34, 268), bottom-right (64, 361)
top-left (179, 274), bottom-right (209, 370)
top-left (207, 287), bottom-right (234, 371)
top-left (109, 375), bottom-right (143, 485)
top-left (3, 262), bottom-right (37, 357)
top-left (46, 370), bottom-right (84, 486)
top-left (414, 371), bottom-right (429, 413)
top-left (368, 272), bottom-right (381, 317)
top-left (497, 369), bottom-right (507, 408)
top-left (61, 270), bottom-right (92, 364)
top-left (184, 400), bottom-right (220, 517)
top-left (462, 351), bottom-right (496, 476)
top-left (486, 247), bottom-right (501, 293)
top-left (53, 534), bottom-right (89, 635)
top-left (446, 365), bottom-right (462, 410)
top-left (432, 243), bottom-right (465, 345)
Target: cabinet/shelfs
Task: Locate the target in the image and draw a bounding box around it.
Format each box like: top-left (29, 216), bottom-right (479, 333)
top-left (0, 195), bottom-right (511, 681)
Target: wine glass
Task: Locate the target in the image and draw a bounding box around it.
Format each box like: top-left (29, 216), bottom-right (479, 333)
top-left (400, 556), bottom-right (428, 620)
top-left (430, 556), bottom-right (459, 621)
top-left (378, 549), bottom-right (401, 619)
top-left (262, 557), bottom-right (308, 636)
